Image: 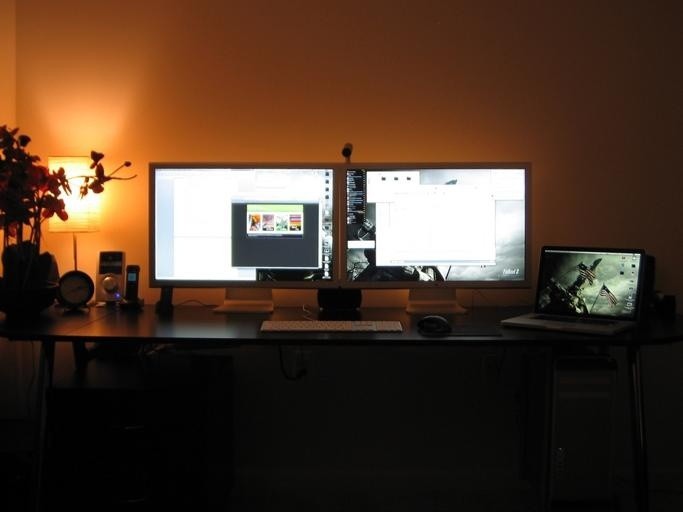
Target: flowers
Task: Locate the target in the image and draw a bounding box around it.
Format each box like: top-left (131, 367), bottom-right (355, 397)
top-left (0, 124), bottom-right (138, 289)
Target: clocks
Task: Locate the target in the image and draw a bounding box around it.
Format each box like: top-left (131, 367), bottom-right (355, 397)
top-left (55, 270), bottom-right (94, 313)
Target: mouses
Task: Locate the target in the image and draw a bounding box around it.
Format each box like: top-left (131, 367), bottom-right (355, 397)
top-left (418, 316), bottom-right (451, 333)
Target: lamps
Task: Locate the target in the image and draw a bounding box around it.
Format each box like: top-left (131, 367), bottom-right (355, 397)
top-left (47, 158), bottom-right (103, 309)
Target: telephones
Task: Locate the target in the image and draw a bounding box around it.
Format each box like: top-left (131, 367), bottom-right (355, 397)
top-left (119, 265), bottom-right (144, 310)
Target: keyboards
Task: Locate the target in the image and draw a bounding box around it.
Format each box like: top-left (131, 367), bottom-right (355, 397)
top-left (261, 320), bottom-right (404, 332)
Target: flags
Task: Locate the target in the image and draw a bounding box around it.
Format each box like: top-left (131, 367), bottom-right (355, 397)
top-left (598, 286), bottom-right (616, 304)
top-left (573, 263), bottom-right (595, 286)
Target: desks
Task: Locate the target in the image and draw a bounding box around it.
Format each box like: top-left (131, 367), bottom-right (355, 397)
top-left (1, 306), bottom-right (683, 512)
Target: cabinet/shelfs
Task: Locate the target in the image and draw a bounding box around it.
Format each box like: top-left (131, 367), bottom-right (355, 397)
top-left (41, 353), bottom-right (234, 512)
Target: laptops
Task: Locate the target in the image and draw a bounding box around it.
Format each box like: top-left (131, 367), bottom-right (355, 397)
top-left (500, 246), bottom-right (645, 334)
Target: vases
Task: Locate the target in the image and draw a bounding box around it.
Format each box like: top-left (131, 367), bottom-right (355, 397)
top-left (0, 279), bottom-right (57, 314)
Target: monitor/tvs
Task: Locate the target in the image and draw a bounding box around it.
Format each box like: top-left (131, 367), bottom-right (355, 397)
top-left (149, 162), bottom-right (338, 312)
top-left (338, 162), bottom-right (531, 315)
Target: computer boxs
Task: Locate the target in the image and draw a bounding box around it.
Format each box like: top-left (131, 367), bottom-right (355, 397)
top-left (159, 341), bottom-right (233, 512)
top-left (522, 350), bottom-right (617, 512)
